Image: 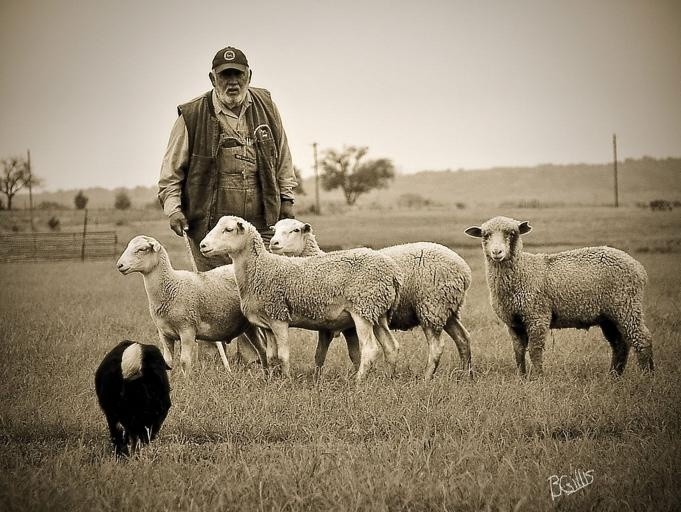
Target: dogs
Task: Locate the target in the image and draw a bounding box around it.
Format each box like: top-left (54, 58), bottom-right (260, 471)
top-left (116, 234), bottom-right (267, 380)
top-left (94, 339), bottom-right (173, 457)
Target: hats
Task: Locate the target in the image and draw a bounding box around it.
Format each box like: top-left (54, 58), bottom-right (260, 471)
top-left (212, 46), bottom-right (249, 74)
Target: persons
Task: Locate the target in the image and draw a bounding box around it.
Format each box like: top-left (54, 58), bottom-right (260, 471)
top-left (157, 46), bottom-right (300, 367)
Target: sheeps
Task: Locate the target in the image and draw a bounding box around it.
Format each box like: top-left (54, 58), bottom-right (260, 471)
top-left (199, 215), bottom-right (402, 382)
top-left (268, 217), bottom-right (473, 383)
top-left (464, 215), bottom-right (656, 378)
